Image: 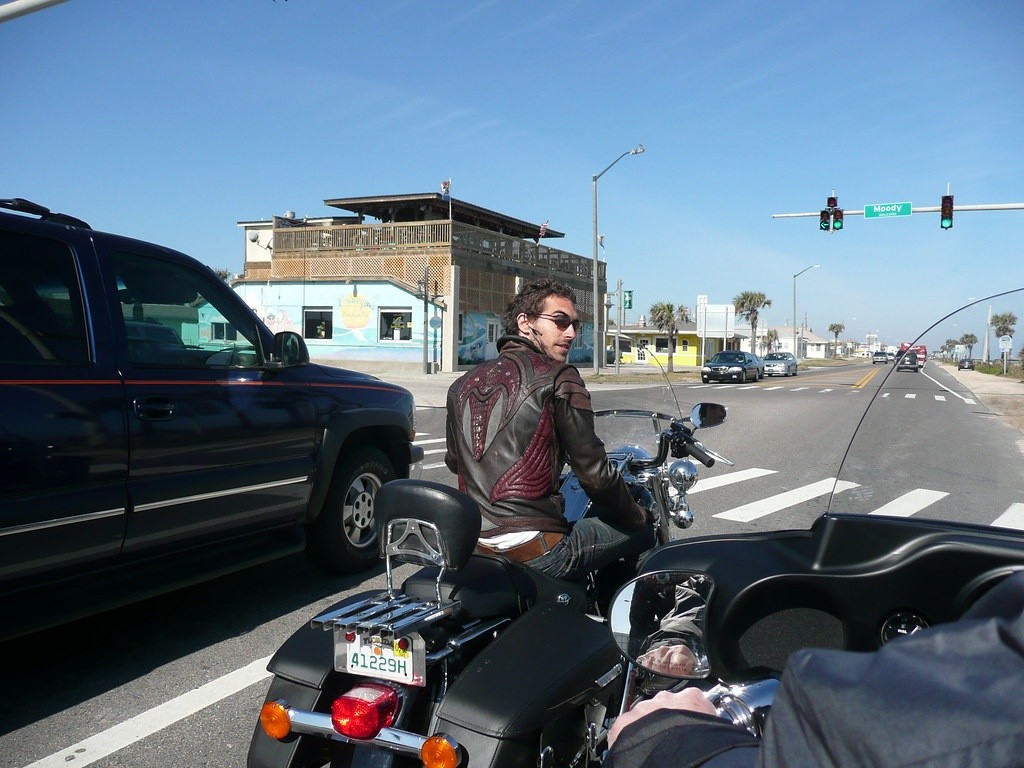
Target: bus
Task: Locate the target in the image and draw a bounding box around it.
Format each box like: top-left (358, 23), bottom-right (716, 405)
top-left (900, 341), bottom-right (927, 368)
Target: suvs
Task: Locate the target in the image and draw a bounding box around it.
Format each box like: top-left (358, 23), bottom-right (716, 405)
top-left (1, 190), bottom-right (416, 655)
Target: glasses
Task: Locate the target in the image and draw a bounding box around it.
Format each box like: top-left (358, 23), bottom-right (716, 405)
top-left (530, 312), bottom-right (580, 332)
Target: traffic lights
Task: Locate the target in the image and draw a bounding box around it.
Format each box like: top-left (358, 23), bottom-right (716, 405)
top-left (940, 195), bottom-right (954, 228)
top-left (827, 197), bottom-right (838, 207)
top-left (820, 210), bottom-right (830, 230)
top-left (832, 208), bottom-right (843, 229)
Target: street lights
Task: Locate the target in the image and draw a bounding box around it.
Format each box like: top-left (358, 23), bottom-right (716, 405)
top-left (969, 296), bottom-right (992, 366)
top-left (591, 144), bottom-right (645, 379)
top-left (791, 264), bottom-right (821, 358)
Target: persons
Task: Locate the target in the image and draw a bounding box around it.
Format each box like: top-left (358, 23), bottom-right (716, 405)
top-left (444, 279), bottom-right (661, 579)
top-left (606, 610), bottom-right (1022, 766)
top-left (732, 355), bottom-right (744, 363)
top-left (636, 573), bottom-right (713, 676)
top-left (699, 402), bottom-right (726, 428)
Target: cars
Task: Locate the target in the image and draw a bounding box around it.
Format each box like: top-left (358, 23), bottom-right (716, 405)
top-left (762, 351), bottom-right (798, 377)
top-left (886, 352), bottom-right (896, 361)
top-left (872, 351), bottom-right (888, 364)
top-left (700, 350), bottom-right (765, 384)
top-left (894, 351), bottom-right (919, 372)
top-left (957, 358), bottom-right (975, 371)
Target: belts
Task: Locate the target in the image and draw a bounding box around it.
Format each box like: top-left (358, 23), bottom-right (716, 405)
top-left (475, 531), bottom-right (565, 563)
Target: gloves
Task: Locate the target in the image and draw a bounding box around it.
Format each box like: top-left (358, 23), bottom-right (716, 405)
top-left (644, 508), bottom-right (656, 525)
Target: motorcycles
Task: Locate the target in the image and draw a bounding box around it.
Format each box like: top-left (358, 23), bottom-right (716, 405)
top-left (243, 289), bottom-right (1023, 767)
